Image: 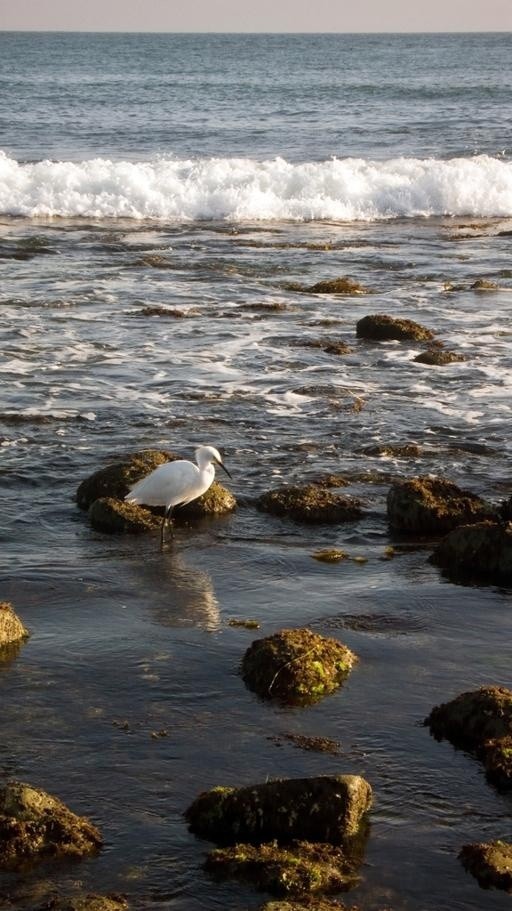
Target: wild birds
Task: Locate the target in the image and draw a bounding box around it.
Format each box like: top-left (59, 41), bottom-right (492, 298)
top-left (122, 445), bottom-right (233, 553)
